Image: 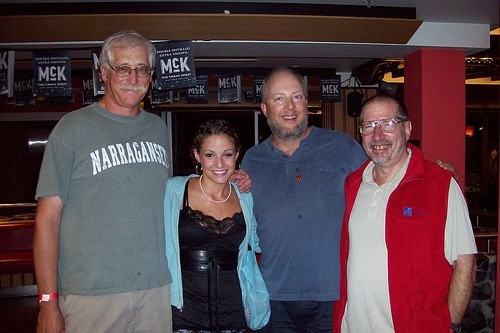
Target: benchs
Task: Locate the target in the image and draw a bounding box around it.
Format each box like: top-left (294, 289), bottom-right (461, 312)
top-left (1, 221), bottom-right (38, 301)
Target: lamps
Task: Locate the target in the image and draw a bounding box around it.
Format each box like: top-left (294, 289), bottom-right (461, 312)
top-left (375, 77), bottom-right (401, 96)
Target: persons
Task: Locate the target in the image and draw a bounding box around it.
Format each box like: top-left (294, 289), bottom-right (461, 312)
top-left (332, 95), bottom-right (478, 333)
top-left (163, 120), bottom-right (271, 333)
top-left (34, 28), bottom-right (253, 333)
top-left (240, 67), bottom-right (459, 333)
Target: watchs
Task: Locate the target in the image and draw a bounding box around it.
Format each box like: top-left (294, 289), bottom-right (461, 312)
top-left (450, 322), bottom-right (462, 333)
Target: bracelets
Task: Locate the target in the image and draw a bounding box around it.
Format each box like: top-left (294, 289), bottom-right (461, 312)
top-left (37, 291), bottom-right (59, 302)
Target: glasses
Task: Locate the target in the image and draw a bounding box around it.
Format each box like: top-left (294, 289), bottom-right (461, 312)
top-left (358, 119), bottom-right (403, 136)
top-left (106, 60), bottom-right (156, 78)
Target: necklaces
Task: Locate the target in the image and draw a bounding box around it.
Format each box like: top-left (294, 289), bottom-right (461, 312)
top-left (199, 175), bottom-right (231, 203)
